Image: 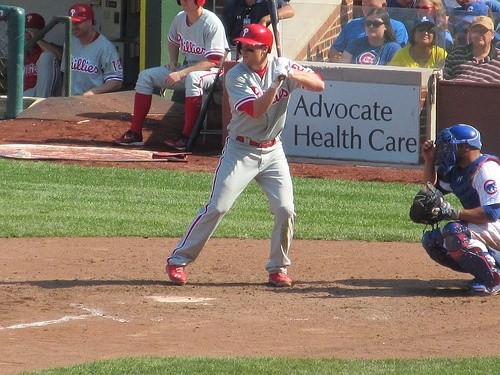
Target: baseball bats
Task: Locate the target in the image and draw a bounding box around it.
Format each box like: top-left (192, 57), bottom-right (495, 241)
top-left (184, 48), bottom-right (233, 152)
top-left (266, 0), bottom-right (289, 82)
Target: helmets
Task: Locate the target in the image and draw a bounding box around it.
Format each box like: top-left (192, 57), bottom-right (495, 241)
top-left (233, 23), bottom-right (273, 53)
top-left (434, 124), bottom-right (482, 176)
top-left (26, 13), bottom-right (45, 30)
top-left (68, 3), bottom-right (94, 24)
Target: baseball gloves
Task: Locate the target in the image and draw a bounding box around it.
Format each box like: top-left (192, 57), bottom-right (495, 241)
top-left (408, 181), bottom-right (460, 227)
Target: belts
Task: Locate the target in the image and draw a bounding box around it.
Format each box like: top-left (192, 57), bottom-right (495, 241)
top-left (236, 135), bottom-right (276, 148)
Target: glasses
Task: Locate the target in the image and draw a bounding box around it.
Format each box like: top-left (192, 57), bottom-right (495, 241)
top-left (415, 5), bottom-right (436, 12)
top-left (415, 27), bottom-right (436, 33)
top-left (364, 19), bottom-right (384, 28)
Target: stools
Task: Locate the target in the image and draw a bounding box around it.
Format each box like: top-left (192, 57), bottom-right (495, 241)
top-left (201, 89), bottom-right (222, 143)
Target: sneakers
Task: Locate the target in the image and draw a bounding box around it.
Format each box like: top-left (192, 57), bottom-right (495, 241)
top-left (470, 277), bottom-right (500, 295)
top-left (166, 262), bottom-right (186, 284)
top-left (115, 130), bottom-right (144, 145)
top-left (269, 272), bottom-right (292, 286)
top-left (163, 135), bottom-right (188, 150)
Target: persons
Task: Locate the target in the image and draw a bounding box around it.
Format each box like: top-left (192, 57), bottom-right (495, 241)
top-left (2, 13), bottom-right (62, 98)
top-left (165, 23), bottom-right (325, 285)
top-left (60, 3), bottom-right (124, 96)
top-left (223, 0), bottom-right (295, 45)
top-left (328, 0), bottom-right (500, 83)
top-left (409, 124), bottom-right (500, 295)
top-left (113, 0), bottom-right (231, 151)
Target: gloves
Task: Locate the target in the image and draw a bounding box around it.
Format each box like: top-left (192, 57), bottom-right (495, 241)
top-left (270, 66), bottom-right (288, 91)
top-left (274, 56), bottom-right (293, 77)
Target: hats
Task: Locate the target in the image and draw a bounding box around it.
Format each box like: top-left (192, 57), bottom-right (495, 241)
top-left (470, 16), bottom-right (494, 34)
top-left (414, 16), bottom-right (436, 27)
top-left (461, 2), bottom-right (487, 23)
